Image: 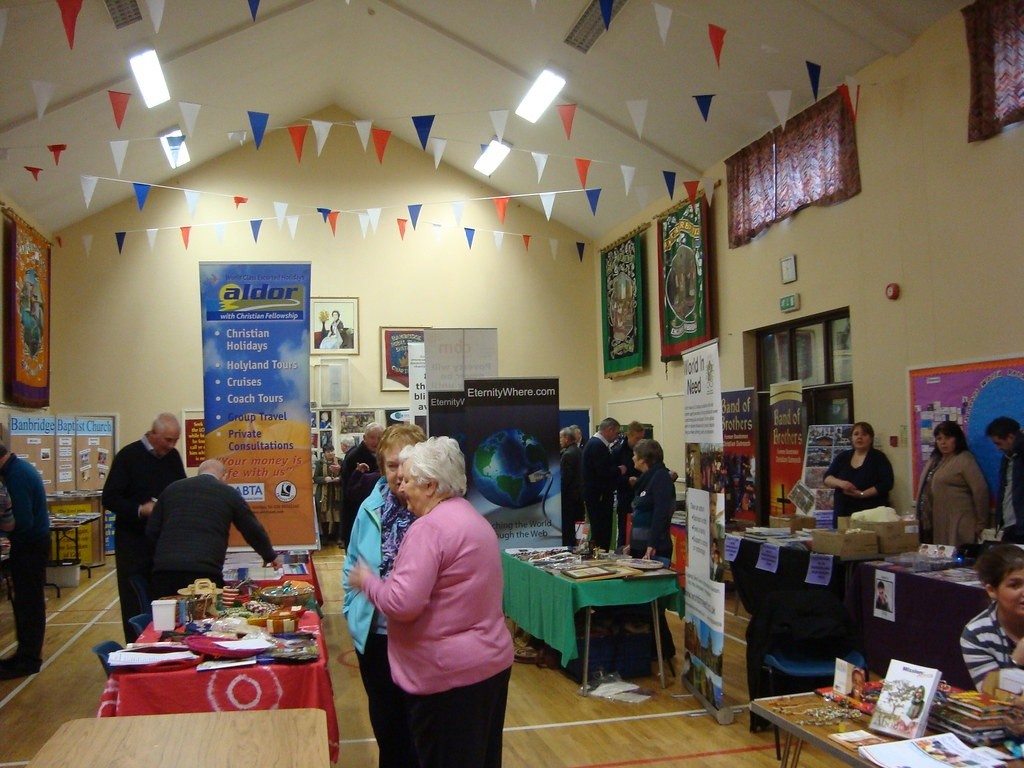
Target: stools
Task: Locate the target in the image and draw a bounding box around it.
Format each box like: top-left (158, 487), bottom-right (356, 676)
top-left (760, 647), bottom-right (865, 759)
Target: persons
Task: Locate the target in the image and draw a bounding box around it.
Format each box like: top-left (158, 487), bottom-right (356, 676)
top-left (313, 444), bottom-right (343, 545)
top-left (907, 686), bottom-right (925, 718)
top-left (102, 414), bottom-right (188, 644)
top-left (915, 422), bottom-right (990, 545)
top-left (846, 670), bottom-right (863, 700)
top-left (985, 416), bottom-right (1024, 545)
top-left (559, 417), bottom-right (676, 660)
top-left (876, 582), bottom-right (889, 610)
top-left (341, 422), bottom-right (385, 554)
top-left (147, 459), bottom-right (282, 597)
top-left (351, 436), bottom-right (514, 767)
top-left (343, 424), bottom-right (429, 768)
top-left (346, 461), bottom-right (382, 508)
top-left (823, 422), bottom-right (893, 527)
top-left (329, 439), bottom-right (356, 549)
top-left (0, 444), bottom-right (52, 679)
top-left (709, 494), bottom-right (725, 581)
top-left (920, 545), bottom-right (946, 557)
top-left (958, 545), bottom-right (1024, 694)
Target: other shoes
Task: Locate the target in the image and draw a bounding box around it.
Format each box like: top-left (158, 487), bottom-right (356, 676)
top-left (339, 543), bottom-right (345, 549)
top-left (0, 652), bottom-right (42, 680)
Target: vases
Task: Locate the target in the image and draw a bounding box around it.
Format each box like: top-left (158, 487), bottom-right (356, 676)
top-left (322, 321), bottom-right (327, 336)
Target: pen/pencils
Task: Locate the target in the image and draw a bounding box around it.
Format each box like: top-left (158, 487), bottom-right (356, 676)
top-left (230, 579), bottom-right (246, 588)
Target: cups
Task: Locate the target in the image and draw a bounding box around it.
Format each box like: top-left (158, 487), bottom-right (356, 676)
top-left (188, 598), bottom-right (208, 620)
top-left (151, 600), bottom-right (176, 631)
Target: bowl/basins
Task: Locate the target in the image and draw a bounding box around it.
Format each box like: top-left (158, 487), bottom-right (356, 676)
top-left (262, 585), bottom-right (313, 608)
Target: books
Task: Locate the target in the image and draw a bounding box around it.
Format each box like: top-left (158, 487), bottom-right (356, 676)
top-left (927, 692), bottom-right (1024, 747)
top-left (870, 659), bottom-right (943, 739)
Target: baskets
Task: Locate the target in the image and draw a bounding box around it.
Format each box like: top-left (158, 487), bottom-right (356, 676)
top-left (259, 585), bottom-right (312, 607)
top-left (159, 594), bottom-right (214, 622)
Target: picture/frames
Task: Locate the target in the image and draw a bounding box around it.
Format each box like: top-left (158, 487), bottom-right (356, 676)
top-left (309, 296), bottom-right (359, 355)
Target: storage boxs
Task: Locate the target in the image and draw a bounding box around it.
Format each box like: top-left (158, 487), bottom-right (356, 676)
top-left (564, 631), bottom-right (655, 683)
top-left (770, 515), bottom-right (920, 560)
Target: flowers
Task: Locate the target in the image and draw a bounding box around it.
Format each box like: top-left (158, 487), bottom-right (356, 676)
top-left (319, 311), bottom-right (329, 323)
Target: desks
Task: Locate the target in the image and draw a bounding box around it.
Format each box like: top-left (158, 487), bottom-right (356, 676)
top-left (846, 560), bottom-right (989, 690)
top-left (748, 691), bottom-right (1024, 767)
top-left (497, 549), bottom-right (684, 691)
top-left (724, 535), bottom-right (858, 728)
top-left (1, 490), bottom-right (339, 768)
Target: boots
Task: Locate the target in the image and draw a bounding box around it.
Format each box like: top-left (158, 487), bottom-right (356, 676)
top-left (334, 522), bottom-right (344, 545)
top-left (320, 522), bottom-right (329, 546)
top-left (657, 619), bottom-right (675, 660)
